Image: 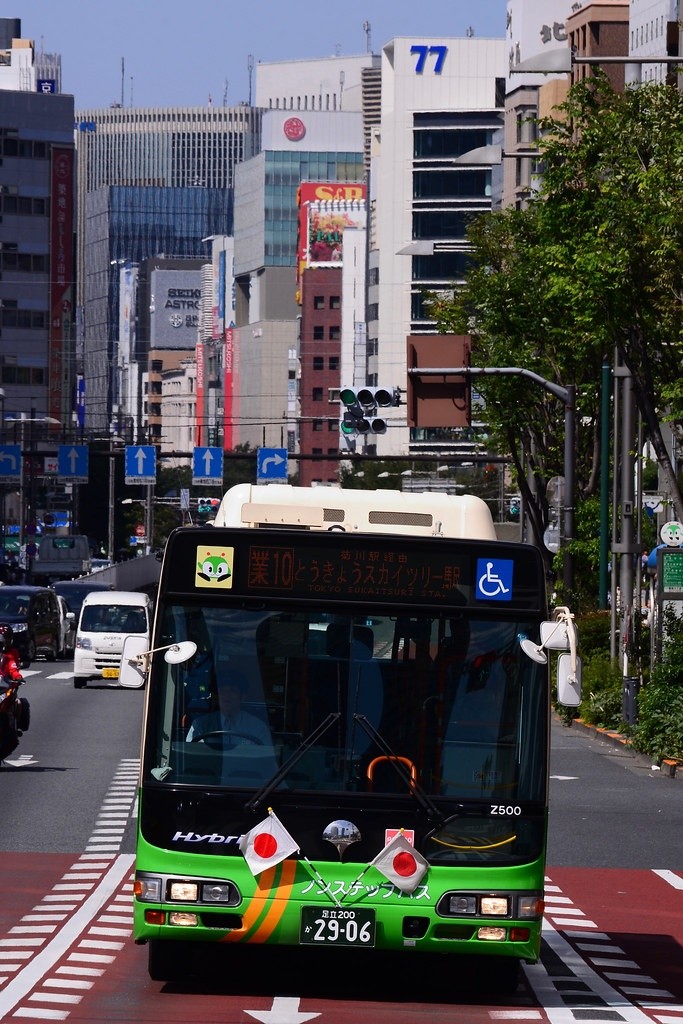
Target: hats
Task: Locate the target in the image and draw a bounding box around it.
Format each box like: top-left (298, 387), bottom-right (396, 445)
top-left (209, 669), bottom-right (248, 694)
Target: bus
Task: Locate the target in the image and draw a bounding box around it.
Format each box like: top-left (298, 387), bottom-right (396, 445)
top-left (117, 485), bottom-right (582, 982)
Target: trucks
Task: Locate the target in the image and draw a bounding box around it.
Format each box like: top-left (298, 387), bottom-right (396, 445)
top-left (30, 534), bottom-right (90, 588)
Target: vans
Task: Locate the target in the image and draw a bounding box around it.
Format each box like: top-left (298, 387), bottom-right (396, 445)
top-left (66, 591), bottom-right (152, 688)
top-left (50, 581), bottom-right (116, 628)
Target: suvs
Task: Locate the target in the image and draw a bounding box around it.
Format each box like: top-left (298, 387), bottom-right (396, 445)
top-left (0, 586), bottom-right (75, 669)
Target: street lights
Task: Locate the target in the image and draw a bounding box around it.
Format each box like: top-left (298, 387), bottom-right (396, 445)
top-left (453, 144), bottom-right (641, 735)
top-left (4, 413), bottom-right (60, 564)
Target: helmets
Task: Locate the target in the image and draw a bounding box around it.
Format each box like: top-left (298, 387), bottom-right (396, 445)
top-left (0, 623), bottom-right (13, 651)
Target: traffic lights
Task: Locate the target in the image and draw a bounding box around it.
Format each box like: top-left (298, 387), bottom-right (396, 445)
top-left (199, 498), bottom-right (219, 505)
top-left (340, 385), bottom-right (396, 409)
top-left (197, 506), bottom-right (219, 515)
top-left (338, 419), bottom-right (386, 440)
top-left (510, 497), bottom-right (519, 515)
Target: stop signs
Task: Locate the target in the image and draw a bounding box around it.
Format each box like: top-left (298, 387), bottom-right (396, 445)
top-left (137, 524), bottom-right (145, 536)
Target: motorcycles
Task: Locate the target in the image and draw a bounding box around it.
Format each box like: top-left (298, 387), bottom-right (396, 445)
top-left (0, 657), bottom-right (26, 749)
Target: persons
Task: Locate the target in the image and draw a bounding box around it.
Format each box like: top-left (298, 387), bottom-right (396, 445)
top-left (185, 669), bottom-right (271, 747)
top-left (0, 623), bottom-right (26, 722)
top-left (26, 539), bottom-right (36, 569)
